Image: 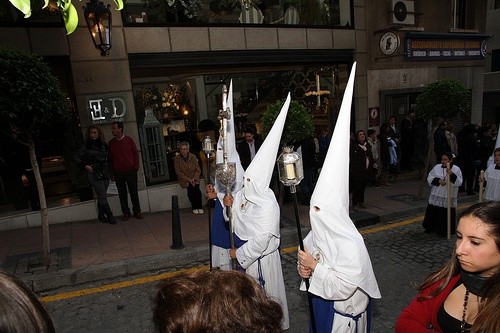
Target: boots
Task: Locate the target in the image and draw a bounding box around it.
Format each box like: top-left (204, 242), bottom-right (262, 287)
top-left (380, 171), bottom-right (392, 186)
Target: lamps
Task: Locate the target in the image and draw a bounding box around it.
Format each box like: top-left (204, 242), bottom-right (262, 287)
top-left (83, 0), bottom-right (112, 58)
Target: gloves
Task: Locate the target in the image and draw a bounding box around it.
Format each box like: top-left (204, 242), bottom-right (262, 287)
top-left (373, 162), bottom-right (378, 168)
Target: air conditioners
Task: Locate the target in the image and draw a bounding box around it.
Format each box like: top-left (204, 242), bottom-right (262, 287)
top-left (389, 0), bottom-right (415, 24)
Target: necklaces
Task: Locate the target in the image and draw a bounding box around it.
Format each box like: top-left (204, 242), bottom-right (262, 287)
top-left (462, 289), bottom-right (469, 322)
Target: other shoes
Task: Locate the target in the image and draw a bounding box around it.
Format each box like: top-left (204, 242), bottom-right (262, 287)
top-left (134, 213), bottom-right (144, 219)
top-left (193, 209), bottom-right (204, 214)
top-left (467, 191), bottom-right (477, 195)
top-left (122, 212), bottom-right (130, 220)
top-left (376, 185), bottom-right (380, 189)
top-left (359, 204), bottom-right (367, 209)
top-left (98, 215), bottom-right (108, 223)
top-left (351, 206), bottom-right (362, 212)
top-left (108, 217), bottom-right (117, 225)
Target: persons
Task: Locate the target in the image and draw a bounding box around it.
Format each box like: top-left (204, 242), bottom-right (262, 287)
top-left (174, 143), bottom-right (203, 214)
top-left (428, 121), bottom-right (500, 195)
top-left (72, 126), bottom-right (117, 224)
top-left (297, 110), bottom-right (420, 212)
top-left (237, 129), bottom-right (262, 172)
top-left (422, 152), bottom-right (463, 237)
top-left (0, 267), bottom-right (56, 333)
top-left (152, 265), bottom-right (285, 333)
top-left (395, 199), bottom-right (500, 333)
top-left (204, 133), bottom-right (382, 333)
top-left (478, 147), bottom-right (500, 200)
top-left (107, 121), bottom-right (145, 220)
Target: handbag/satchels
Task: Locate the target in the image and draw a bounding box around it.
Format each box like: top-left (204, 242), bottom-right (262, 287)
top-left (92, 165), bottom-right (105, 181)
